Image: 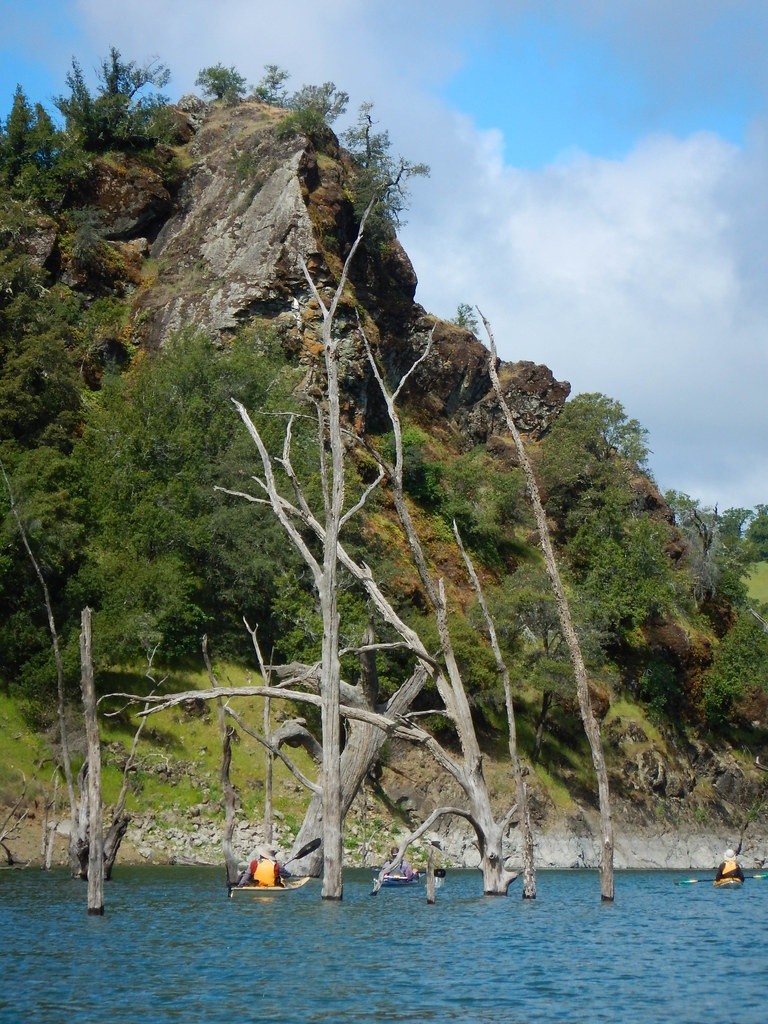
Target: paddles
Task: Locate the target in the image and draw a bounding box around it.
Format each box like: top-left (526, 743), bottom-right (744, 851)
top-left (674, 872), bottom-right (768, 888)
top-left (366, 862), bottom-right (447, 880)
top-left (278, 837), bottom-right (325, 866)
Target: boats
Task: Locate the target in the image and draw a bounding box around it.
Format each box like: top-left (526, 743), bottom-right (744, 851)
top-left (231, 876), bottom-right (311, 891)
top-left (383, 874), bottom-right (419, 885)
top-left (712, 877), bottom-right (743, 889)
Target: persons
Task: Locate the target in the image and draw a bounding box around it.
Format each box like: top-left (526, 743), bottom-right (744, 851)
top-left (716, 849), bottom-right (745, 882)
top-left (240, 848), bottom-right (284, 886)
top-left (382, 846), bottom-right (413, 877)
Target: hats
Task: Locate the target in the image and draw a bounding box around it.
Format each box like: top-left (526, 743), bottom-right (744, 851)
top-left (724, 849), bottom-right (736, 861)
top-left (258, 844), bottom-right (278, 861)
